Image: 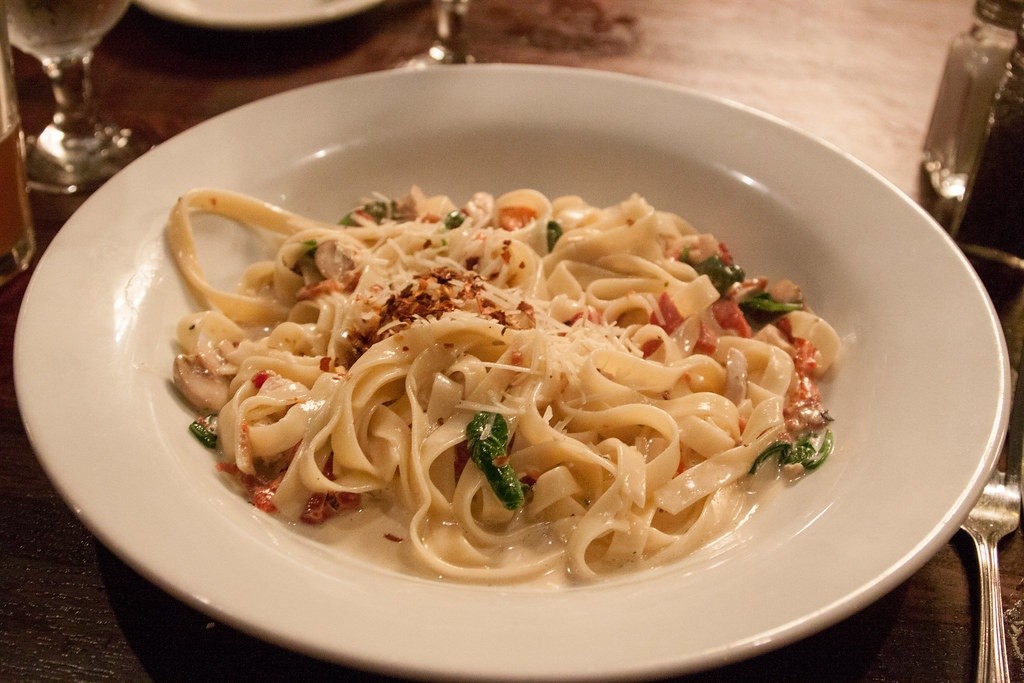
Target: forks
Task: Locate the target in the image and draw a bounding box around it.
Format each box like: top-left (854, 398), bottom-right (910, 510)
top-left (935, 364), bottom-right (1021, 683)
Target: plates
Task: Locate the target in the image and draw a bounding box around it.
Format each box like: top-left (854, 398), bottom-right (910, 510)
top-left (12, 59), bottom-right (1012, 683)
top-left (138, 0), bottom-right (386, 32)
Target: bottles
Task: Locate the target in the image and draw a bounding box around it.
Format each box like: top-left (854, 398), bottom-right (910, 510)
top-left (920, 0), bottom-right (1021, 198)
top-left (948, 21), bottom-right (1024, 272)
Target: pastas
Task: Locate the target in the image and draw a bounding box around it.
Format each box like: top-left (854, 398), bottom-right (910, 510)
top-left (168, 188), bottom-right (843, 587)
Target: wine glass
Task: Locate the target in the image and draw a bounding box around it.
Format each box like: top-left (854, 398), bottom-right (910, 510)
top-left (396, 0), bottom-right (486, 86)
top-left (0, 0), bottom-right (159, 192)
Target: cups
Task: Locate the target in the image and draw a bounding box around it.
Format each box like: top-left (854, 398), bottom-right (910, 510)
top-left (0, 6), bottom-right (39, 293)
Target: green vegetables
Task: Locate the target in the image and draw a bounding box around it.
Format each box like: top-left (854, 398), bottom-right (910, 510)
top-left (193, 197), bottom-right (835, 514)
